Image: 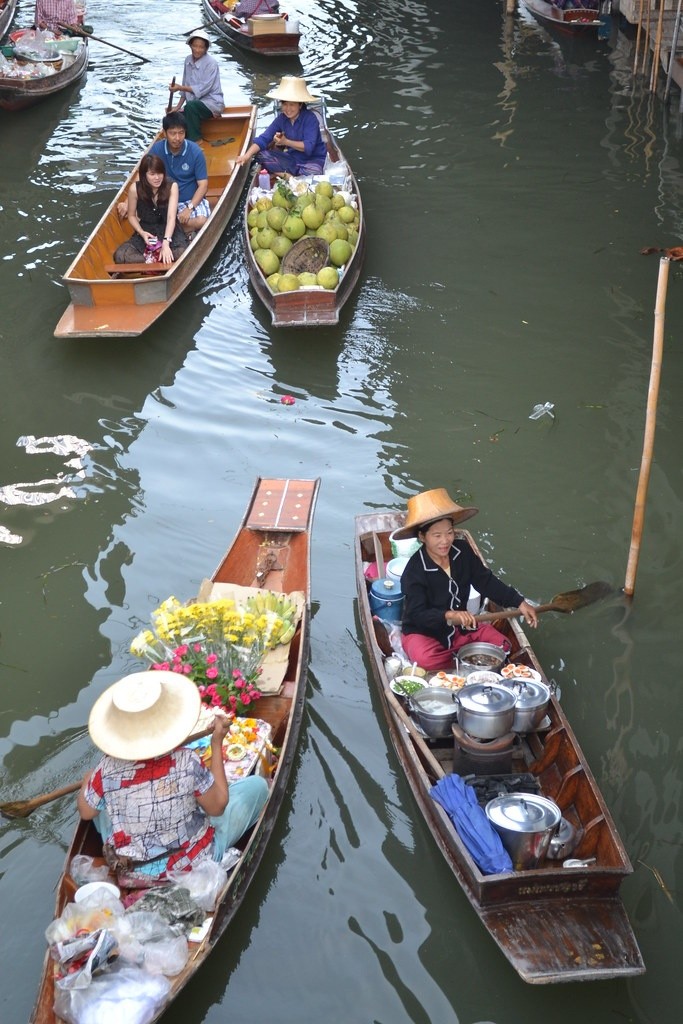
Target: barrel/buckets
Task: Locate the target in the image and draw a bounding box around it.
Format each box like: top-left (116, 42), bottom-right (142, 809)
top-left (467, 584), bottom-right (481, 615)
top-left (74, 882), bottom-right (120, 903)
top-left (385, 556), bottom-right (410, 581)
top-left (9, 32), bottom-right (35, 44)
top-left (368, 579), bottom-right (405, 621)
top-left (285, 21), bottom-right (299, 33)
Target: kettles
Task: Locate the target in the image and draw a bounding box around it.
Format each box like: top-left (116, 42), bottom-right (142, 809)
top-left (545, 795), bottom-right (576, 859)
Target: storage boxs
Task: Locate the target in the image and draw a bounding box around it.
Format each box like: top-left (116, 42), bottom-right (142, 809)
top-left (246, 17), bottom-right (285, 36)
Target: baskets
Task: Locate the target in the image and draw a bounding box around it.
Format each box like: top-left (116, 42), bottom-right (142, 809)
top-left (281, 236), bottom-right (330, 276)
top-left (46, 40), bottom-right (80, 52)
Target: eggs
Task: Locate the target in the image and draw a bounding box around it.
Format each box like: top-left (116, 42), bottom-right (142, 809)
top-left (438, 664), bottom-right (531, 690)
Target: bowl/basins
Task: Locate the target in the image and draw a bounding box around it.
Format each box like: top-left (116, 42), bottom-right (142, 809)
top-left (46, 40), bottom-right (80, 51)
top-left (403, 667), bottom-right (426, 677)
top-left (15, 48), bottom-right (62, 61)
top-left (1, 46), bottom-right (15, 56)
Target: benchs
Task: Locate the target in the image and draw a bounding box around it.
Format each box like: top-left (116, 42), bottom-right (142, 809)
top-left (206, 188), bottom-right (223, 197)
top-left (107, 263), bottom-right (177, 273)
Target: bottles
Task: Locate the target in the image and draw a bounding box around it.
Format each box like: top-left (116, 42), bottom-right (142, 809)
top-left (385, 657), bottom-right (402, 682)
top-left (259, 169), bottom-right (270, 190)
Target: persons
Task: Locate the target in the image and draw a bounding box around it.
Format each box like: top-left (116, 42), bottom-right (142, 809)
top-left (32, 0), bottom-right (93, 38)
top-left (235, 77), bottom-right (327, 183)
top-left (389, 489), bottom-right (540, 672)
top-left (76, 670), bottom-right (269, 897)
top-left (229, 0), bottom-right (289, 25)
top-left (111, 111), bottom-right (211, 275)
top-left (164, 30), bottom-right (226, 145)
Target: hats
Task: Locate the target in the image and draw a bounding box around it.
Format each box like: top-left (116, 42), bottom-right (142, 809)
top-left (266, 77), bottom-right (319, 102)
top-left (186, 30), bottom-right (212, 47)
top-left (391, 487), bottom-right (479, 540)
top-left (88, 670), bottom-right (202, 762)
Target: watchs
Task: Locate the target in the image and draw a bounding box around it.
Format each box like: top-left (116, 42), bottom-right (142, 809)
top-left (163, 236), bottom-right (172, 242)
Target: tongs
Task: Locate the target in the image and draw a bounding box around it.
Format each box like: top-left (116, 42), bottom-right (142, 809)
top-left (568, 857), bottom-right (596, 866)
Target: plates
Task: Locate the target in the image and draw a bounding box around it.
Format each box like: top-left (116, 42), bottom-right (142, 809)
top-left (252, 14), bottom-right (282, 21)
top-left (429, 674), bottom-right (461, 689)
top-left (467, 671), bottom-right (503, 684)
top-left (501, 667), bottom-right (541, 681)
top-left (389, 676), bottom-right (429, 696)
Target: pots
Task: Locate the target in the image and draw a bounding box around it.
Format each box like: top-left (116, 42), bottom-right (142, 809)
top-left (452, 639), bottom-right (512, 671)
top-left (452, 682), bottom-right (522, 739)
top-left (485, 791), bottom-right (562, 871)
top-left (408, 687), bottom-right (458, 738)
top-left (497, 678), bottom-right (558, 732)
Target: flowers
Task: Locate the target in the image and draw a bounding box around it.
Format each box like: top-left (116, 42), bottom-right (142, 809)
top-left (128, 596), bottom-right (283, 717)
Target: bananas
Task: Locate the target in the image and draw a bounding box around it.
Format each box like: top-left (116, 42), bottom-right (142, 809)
top-left (247, 590), bottom-right (297, 645)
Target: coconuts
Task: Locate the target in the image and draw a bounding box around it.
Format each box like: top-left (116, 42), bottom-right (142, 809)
top-left (247, 181), bottom-right (361, 291)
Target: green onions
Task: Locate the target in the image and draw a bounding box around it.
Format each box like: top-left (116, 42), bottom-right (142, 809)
top-left (393, 679), bottom-right (425, 695)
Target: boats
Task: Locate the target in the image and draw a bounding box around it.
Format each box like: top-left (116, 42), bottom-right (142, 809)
top-left (524, 1), bottom-right (605, 26)
top-left (243, 93), bottom-right (365, 325)
top-left (202, 0), bottom-right (304, 56)
top-left (27, 477), bottom-right (306, 1023)
top-left (355, 510), bottom-right (649, 985)
top-left (53, 106), bottom-right (260, 338)
top-left (0, 23), bottom-right (90, 109)
